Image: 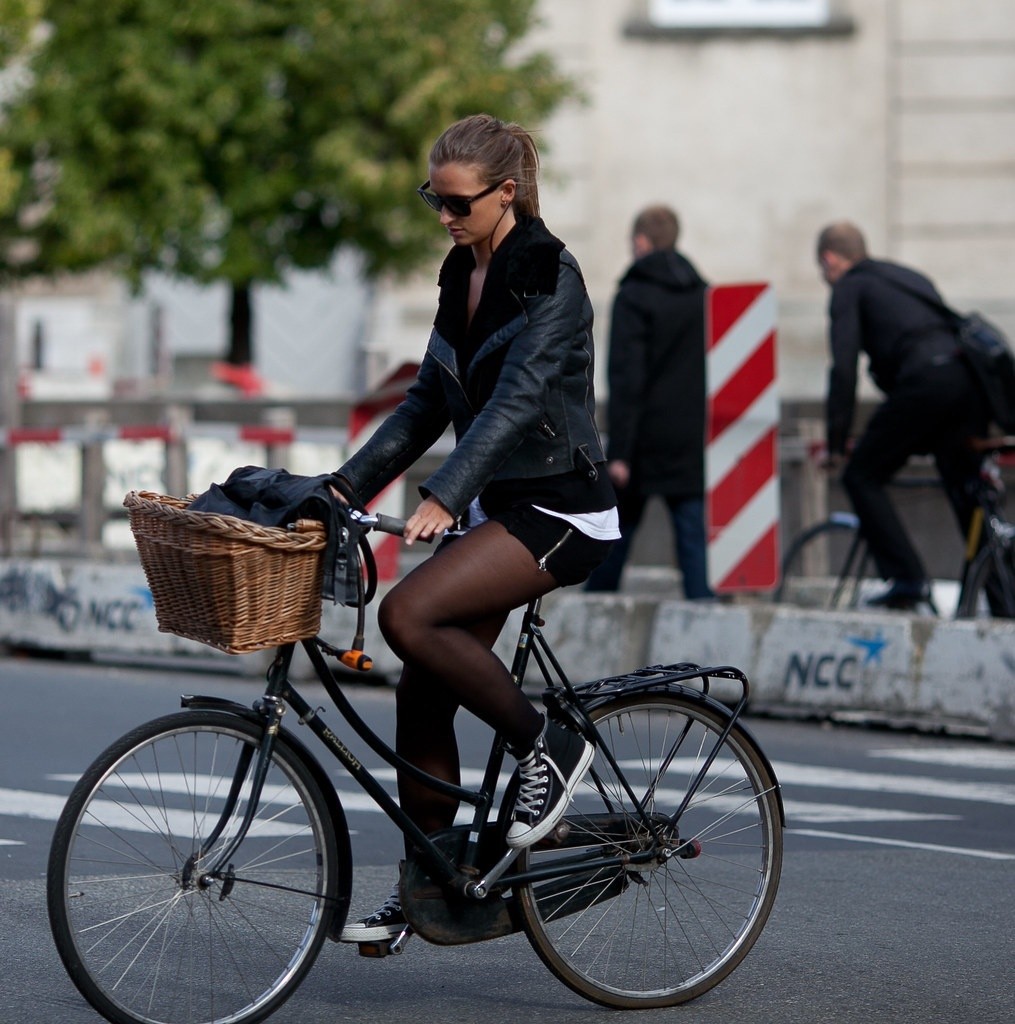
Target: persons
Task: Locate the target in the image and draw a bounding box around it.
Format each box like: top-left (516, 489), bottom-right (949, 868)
top-left (328, 112), bottom-right (626, 943)
top-left (582, 204), bottom-right (733, 601)
top-left (816, 223), bottom-right (1015, 619)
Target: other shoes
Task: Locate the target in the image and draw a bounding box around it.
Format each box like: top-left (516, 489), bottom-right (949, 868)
top-left (868, 578), bottom-right (930, 606)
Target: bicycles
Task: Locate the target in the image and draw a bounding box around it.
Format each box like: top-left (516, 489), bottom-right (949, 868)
top-left (46, 508), bottom-right (786, 1024)
top-left (774, 428), bottom-right (1014, 621)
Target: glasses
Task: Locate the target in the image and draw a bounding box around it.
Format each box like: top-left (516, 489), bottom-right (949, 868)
top-left (417, 179), bottom-right (510, 216)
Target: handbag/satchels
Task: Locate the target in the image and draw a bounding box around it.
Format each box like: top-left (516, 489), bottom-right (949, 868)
top-left (960, 313), bottom-right (1015, 435)
top-left (188, 465), bottom-right (377, 607)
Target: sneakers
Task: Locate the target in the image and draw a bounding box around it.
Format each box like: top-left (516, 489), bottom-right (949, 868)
top-left (338, 883), bottom-right (412, 943)
top-left (506, 711), bottom-right (596, 848)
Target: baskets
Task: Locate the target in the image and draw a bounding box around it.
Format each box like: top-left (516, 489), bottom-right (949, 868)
top-left (125, 488), bottom-right (330, 656)
